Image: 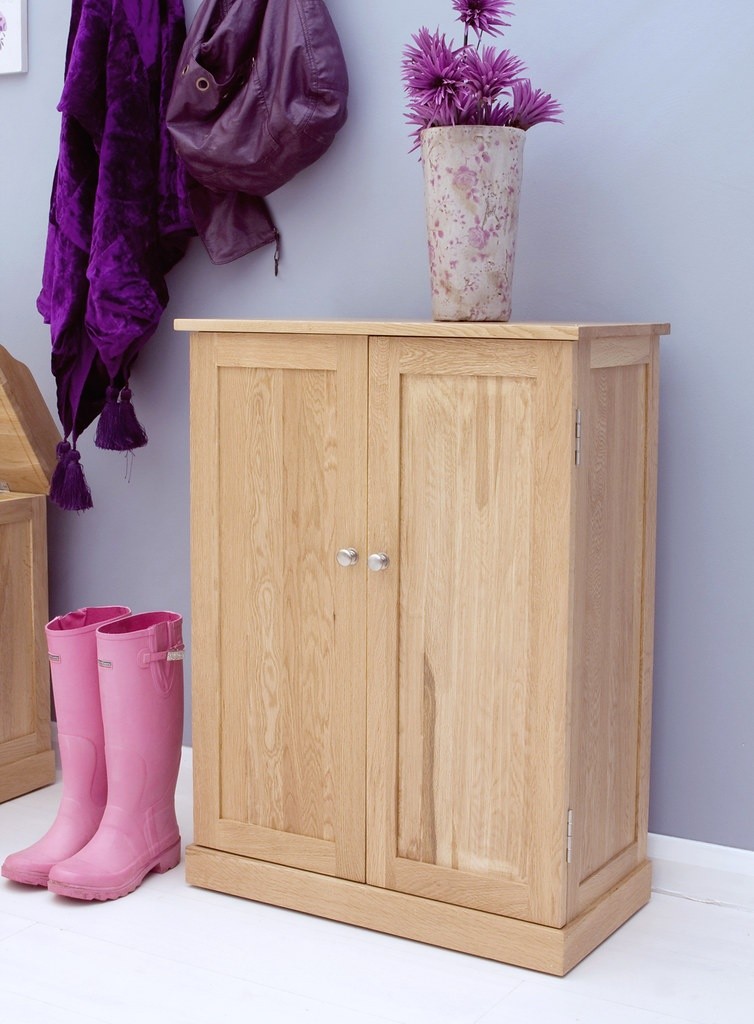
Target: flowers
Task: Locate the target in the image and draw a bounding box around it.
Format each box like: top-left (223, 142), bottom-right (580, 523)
top-left (399, 0), bottom-right (566, 164)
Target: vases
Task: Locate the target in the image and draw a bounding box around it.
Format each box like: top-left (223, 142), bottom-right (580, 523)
top-left (419, 125), bottom-right (528, 322)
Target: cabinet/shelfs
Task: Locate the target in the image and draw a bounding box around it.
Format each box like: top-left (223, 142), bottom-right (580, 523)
top-left (170, 317), bottom-right (675, 980)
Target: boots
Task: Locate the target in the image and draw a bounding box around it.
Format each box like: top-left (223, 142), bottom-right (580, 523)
top-left (3, 604), bottom-right (134, 889)
top-left (48, 610), bottom-right (185, 901)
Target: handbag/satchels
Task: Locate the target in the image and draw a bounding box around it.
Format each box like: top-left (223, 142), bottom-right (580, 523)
top-left (167, 0), bottom-right (348, 275)
